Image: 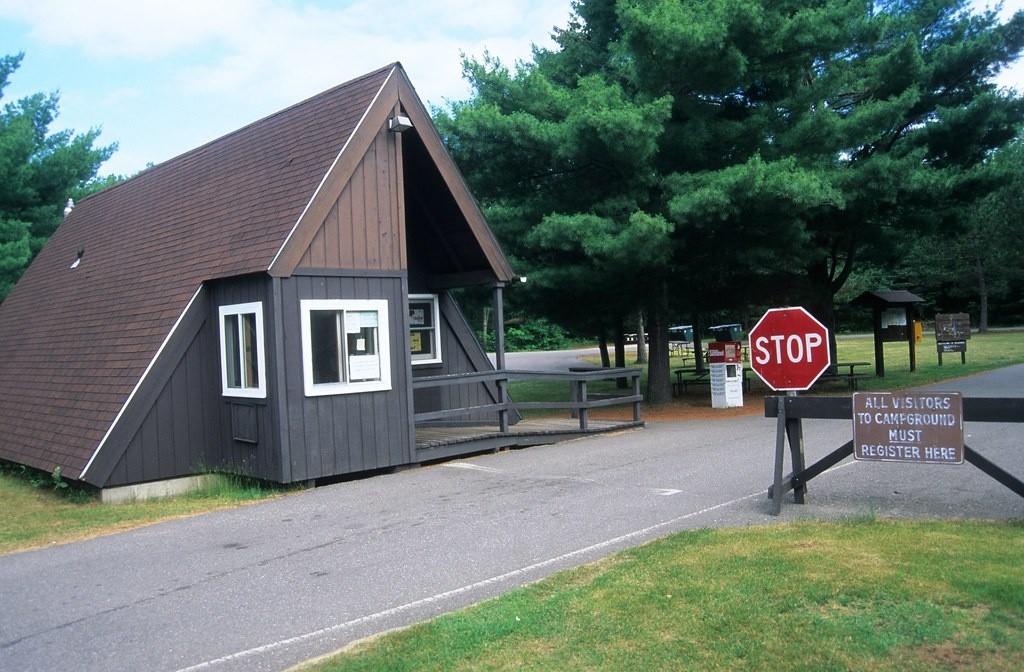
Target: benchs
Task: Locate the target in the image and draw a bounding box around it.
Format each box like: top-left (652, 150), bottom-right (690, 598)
top-left (668, 347), bottom-right (874, 399)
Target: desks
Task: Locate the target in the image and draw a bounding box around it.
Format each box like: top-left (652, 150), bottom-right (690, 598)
top-left (669, 341), bottom-right (691, 357)
top-left (674, 367), bottom-right (753, 397)
top-left (829, 361), bottom-right (871, 388)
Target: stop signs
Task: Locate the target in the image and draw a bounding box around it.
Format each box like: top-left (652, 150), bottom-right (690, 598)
top-left (748, 305), bottom-right (830, 391)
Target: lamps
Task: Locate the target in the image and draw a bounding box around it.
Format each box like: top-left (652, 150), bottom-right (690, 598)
top-left (387, 115), bottom-right (413, 133)
